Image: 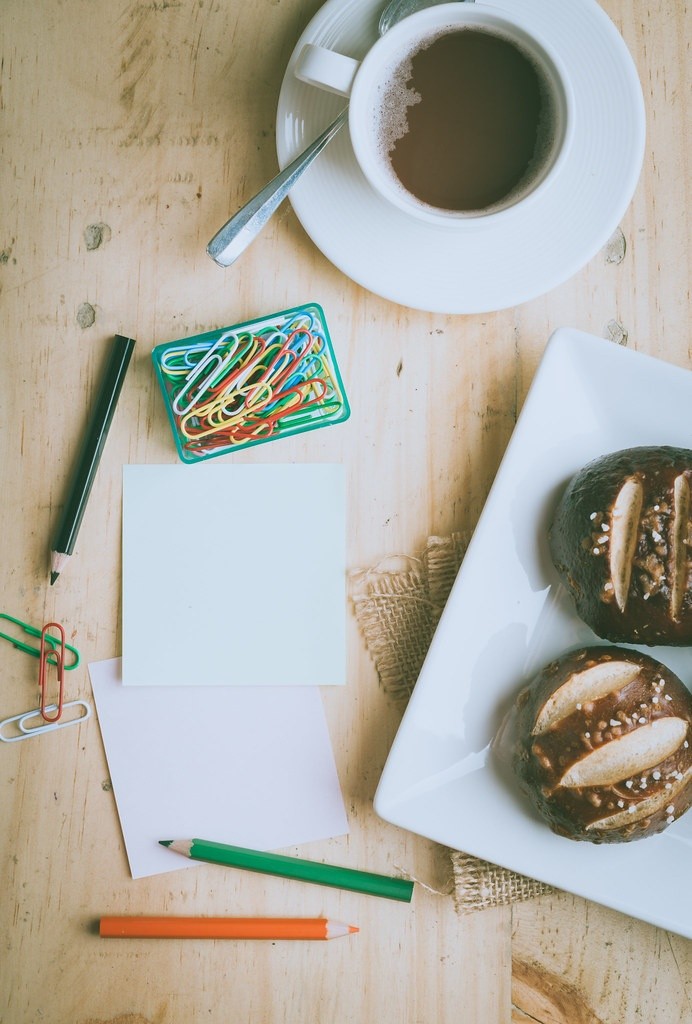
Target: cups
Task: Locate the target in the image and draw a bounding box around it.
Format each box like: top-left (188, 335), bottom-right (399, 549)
top-left (292, 3), bottom-right (571, 223)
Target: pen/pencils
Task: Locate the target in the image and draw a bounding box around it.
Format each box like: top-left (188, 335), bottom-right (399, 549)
top-left (98, 915), bottom-right (361, 941)
top-left (48, 333), bottom-right (138, 588)
top-left (158, 837), bottom-right (416, 905)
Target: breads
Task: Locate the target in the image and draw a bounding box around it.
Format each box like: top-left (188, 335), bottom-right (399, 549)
top-left (515, 645), bottom-right (692, 846)
top-left (549, 445), bottom-right (692, 649)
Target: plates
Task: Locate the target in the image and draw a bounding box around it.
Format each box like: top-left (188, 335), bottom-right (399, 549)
top-left (372, 329), bottom-right (692, 939)
top-left (276, 0), bottom-right (646, 313)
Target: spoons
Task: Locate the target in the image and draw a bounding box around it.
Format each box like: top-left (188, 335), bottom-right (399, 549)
top-left (206, 0), bottom-right (475, 267)
